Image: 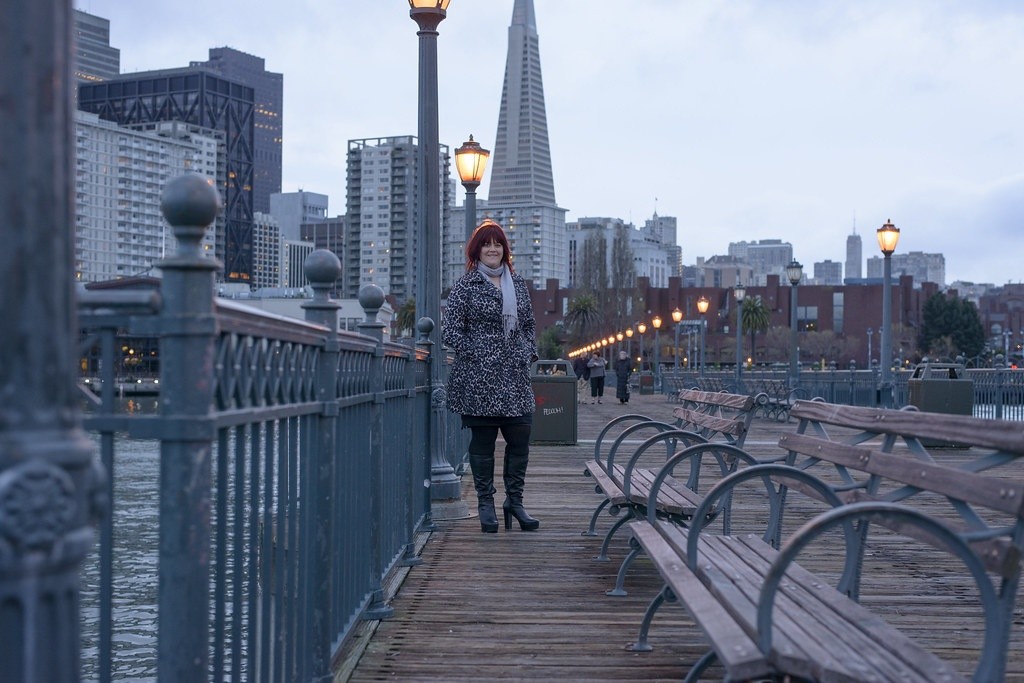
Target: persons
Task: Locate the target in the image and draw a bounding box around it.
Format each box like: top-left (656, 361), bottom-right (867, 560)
top-left (615, 350), bottom-right (633, 404)
top-left (552, 358), bottom-right (568, 376)
top-left (587, 351), bottom-right (608, 404)
top-left (440, 219), bottom-right (541, 531)
top-left (573, 351), bottom-right (590, 404)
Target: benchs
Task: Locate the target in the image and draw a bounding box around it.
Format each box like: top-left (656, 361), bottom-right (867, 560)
top-left (626, 396), bottom-right (1024, 683)
top-left (663, 376), bottom-right (811, 425)
top-left (581, 388), bottom-right (769, 600)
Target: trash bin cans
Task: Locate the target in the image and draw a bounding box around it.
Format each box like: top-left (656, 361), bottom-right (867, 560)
top-left (639, 372), bottom-right (655, 395)
top-left (526, 360), bottom-right (578, 446)
top-left (907, 362), bottom-right (974, 450)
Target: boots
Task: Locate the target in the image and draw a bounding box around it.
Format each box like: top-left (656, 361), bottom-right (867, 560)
top-left (468, 453), bottom-right (498, 532)
top-left (502, 454), bottom-right (540, 530)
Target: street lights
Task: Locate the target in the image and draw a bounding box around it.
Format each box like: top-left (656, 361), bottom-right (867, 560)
top-left (616, 332), bottom-right (624, 352)
top-left (867, 328), bottom-right (873, 370)
top-left (733, 282), bottom-right (746, 393)
top-left (638, 323), bottom-right (646, 370)
top-left (672, 307), bottom-right (683, 376)
top-left (1003, 328), bottom-right (1013, 366)
top-left (608, 335), bottom-right (615, 370)
top-left (626, 327), bottom-right (633, 358)
top-left (652, 315), bottom-right (662, 383)
top-left (455, 134), bottom-right (491, 246)
top-left (407, 0), bottom-right (450, 347)
top-left (697, 295), bottom-right (709, 377)
top-left (786, 258), bottom-right (803, 396)
top-left (875, 219), bottom-right (900, 408)
top-left (601, 337), bottom-right (608, 359)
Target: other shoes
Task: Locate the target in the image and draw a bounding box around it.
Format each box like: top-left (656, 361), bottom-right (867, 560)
top-left (624, 402), bottom-right (627, 405)
top-left (577, 401), bottom-right (580, 404)
top-left (591, 400), bottom-right (595, 404)
top-left (583, 400), bottom-right (586, 403)
top-left (598, 400), bottom-right (603, 404)
